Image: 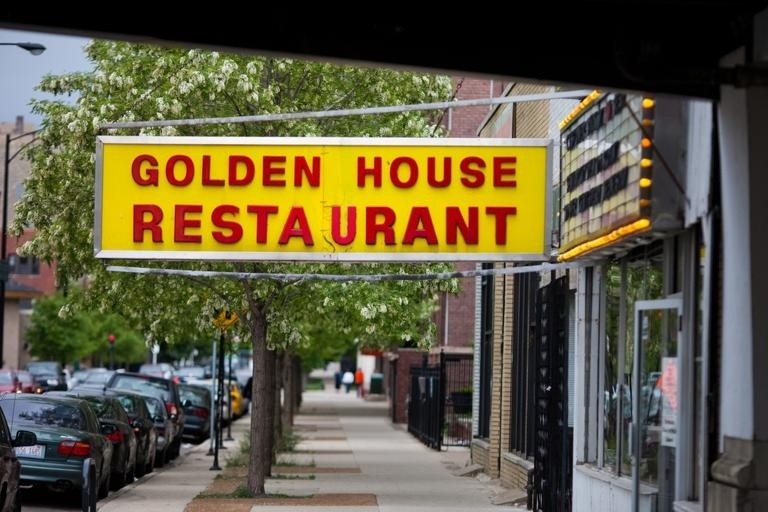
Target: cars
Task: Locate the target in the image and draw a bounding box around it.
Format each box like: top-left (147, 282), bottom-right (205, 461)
top-left (0, 390), bottom-right (116, 512)
top-left (602, 356), bottom-right (703, 501)
top-left (41, 390), bottom-right (145, 492)
top-left (0, 405), bottom-right (37, 512)
top-left (0, 362), bottom-right (253, 477)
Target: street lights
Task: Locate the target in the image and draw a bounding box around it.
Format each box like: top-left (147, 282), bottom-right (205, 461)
top-left (0, 128), bottom-right (70, 369)
top-left (108, 334), bottom-right (115, 370)
top-left (0, 42), bottom-right (46, 56)
top-left (151, 339), bottom-right (160, 364)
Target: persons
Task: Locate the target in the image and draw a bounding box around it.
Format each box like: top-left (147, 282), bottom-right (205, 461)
top-left (334, 367), bottom-right (341, 392)
top-left (355, 367), bottom-right (364, 398)
top-left (243, 376), bottom-right (252, 413)
top-left (342, 368), bottom-right (354, 393)
